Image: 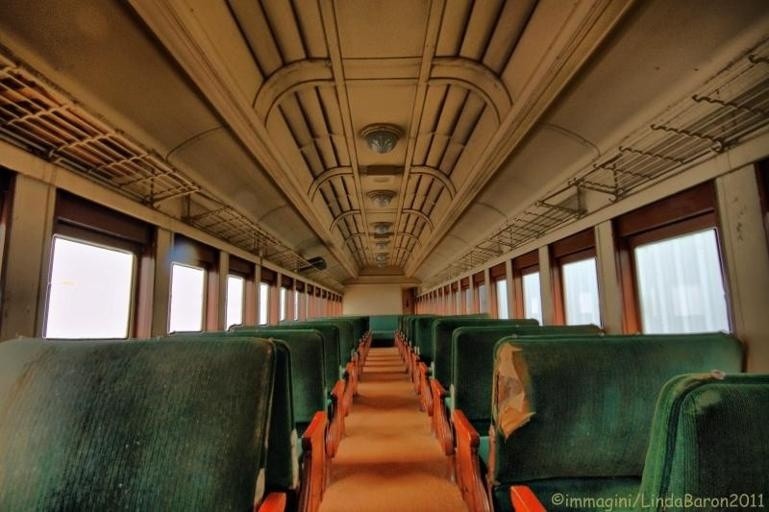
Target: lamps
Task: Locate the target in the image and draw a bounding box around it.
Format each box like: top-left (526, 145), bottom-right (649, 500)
top-left (360, 123), bottom-right (403, 270)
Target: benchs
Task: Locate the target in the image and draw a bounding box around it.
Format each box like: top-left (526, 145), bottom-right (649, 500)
top-left (366, 314), bottom-right (399, 346)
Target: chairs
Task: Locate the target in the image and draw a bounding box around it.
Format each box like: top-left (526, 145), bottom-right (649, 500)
top-left (396, 314), bottom-right (766, 510)
top-left (0, 315), bottom-right (372, 511)
